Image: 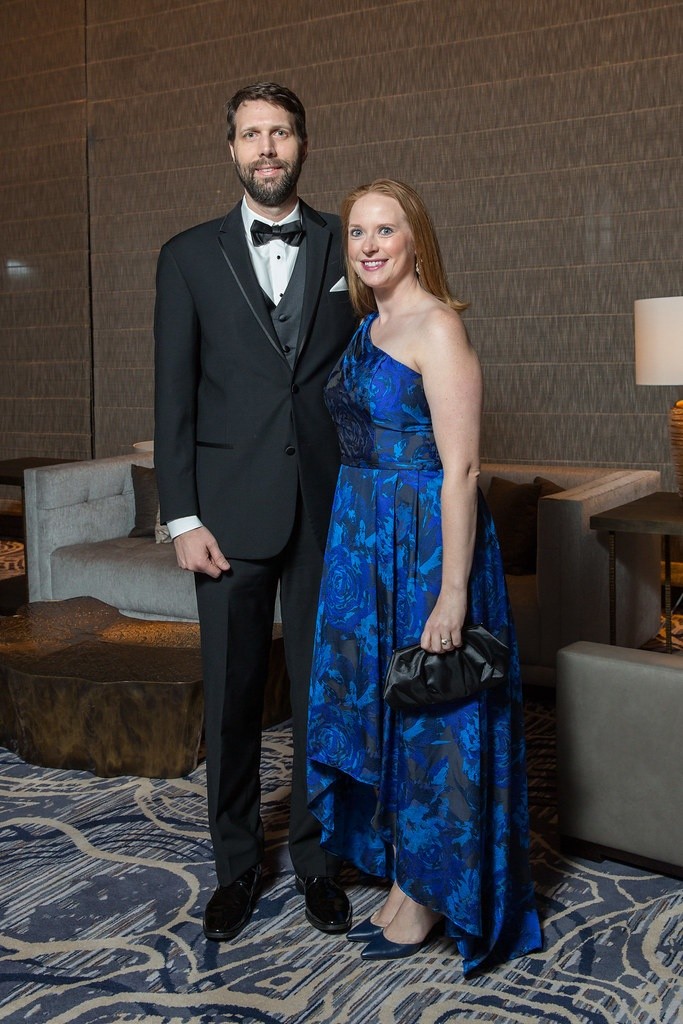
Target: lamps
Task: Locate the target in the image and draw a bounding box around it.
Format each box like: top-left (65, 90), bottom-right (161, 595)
top-left (634, 296), bottom-right (683, 498)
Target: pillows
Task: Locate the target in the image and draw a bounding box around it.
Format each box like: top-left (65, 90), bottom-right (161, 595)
top-left (533, 476), bottom-right (566, 497)
top-left (128, 463), bottom-right (160, 538)
top-left (155, 502), bottom-right (173, 544)
top-left (486, 476), bottom-right (542, 576)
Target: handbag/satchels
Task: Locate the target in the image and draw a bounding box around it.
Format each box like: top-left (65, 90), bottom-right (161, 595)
top-left (383, 626), bottom-right (509, 719)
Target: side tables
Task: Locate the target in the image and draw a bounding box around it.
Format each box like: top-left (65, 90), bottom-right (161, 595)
top-left (0, 457), bottom-right (83, 617)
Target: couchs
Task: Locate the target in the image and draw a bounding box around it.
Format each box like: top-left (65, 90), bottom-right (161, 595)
top-left (555, 640), bottom-right (683, 870)
top-left (24, 452), bottom-right (661, 689)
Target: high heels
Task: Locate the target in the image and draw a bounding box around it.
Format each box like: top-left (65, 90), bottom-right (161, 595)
top-left (346, 910), bottom-right (395, 942)
top-left (361, 911), bottom-right (445, 960)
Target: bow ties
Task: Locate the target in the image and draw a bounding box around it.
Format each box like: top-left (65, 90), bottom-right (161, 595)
top-left (250, 220), bottom-right (303, 246)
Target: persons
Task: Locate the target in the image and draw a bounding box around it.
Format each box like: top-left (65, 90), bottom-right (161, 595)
top-left (155, 82), bottom-right (359, 938)
top-left (301, 177), bottom-right (543, 981)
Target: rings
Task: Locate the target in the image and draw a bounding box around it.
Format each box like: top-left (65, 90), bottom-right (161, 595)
top-left (441, 638), bottom-right (452, 645)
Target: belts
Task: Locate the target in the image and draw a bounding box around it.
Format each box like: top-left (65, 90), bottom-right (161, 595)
top-left (204, 866), bottom-right (261, 941)
top-left (293, 869), bottom-right (351, 932)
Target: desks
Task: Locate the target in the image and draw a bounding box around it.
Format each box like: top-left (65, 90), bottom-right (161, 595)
top-left (0, 596), bottom-right (291, 780)
top-left (589, 492), bottom-right (683, 654)
